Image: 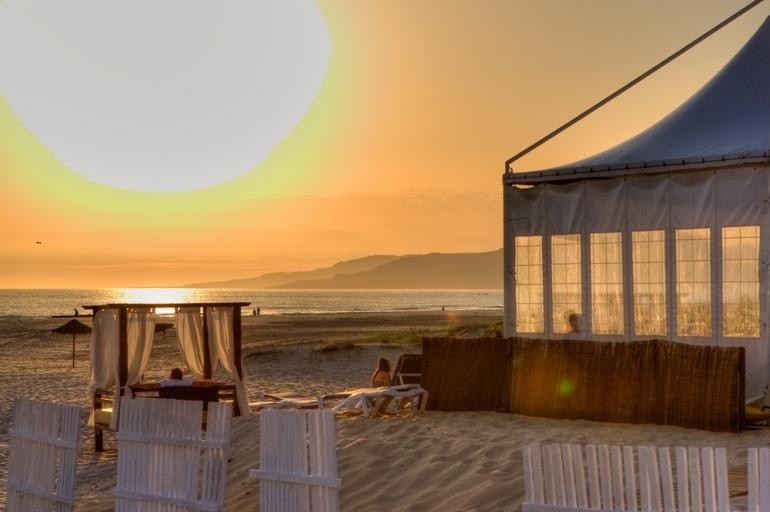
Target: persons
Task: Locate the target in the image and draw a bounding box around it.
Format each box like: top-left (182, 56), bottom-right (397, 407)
top-left (253, 307), bottom-right (260, 316)
top-left (372, 357), bottom-right (392, 388)
top-left (74, 308), bottom-right (78, 316)
top-left (569, 315), bottom-right (581, 333)
top-left (132, 367), bottom-right (225, 390)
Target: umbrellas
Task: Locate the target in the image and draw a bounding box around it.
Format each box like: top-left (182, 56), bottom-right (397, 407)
top-left (53, 319), bottom-right (92, 368)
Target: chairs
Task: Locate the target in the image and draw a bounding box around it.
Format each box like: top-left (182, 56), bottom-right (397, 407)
top-left (247, 382), bottom-right (428, 418)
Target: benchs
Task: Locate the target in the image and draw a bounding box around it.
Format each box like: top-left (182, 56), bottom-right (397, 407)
top-left (94, 386), bottom-right (241, 453)
